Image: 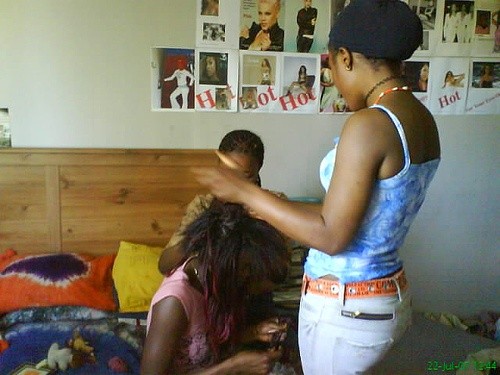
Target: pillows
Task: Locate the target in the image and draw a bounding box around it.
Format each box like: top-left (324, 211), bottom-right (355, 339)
top-left (112, 241), bottom-right (166, 313)
top-left (0, 249), bottom-right (117, 314)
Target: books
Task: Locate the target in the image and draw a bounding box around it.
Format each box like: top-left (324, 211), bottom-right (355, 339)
top-left (271, 246), bottom-right (309, 311)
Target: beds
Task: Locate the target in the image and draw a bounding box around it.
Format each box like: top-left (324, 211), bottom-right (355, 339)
top-left (0, 148), bottom-right (258, 375)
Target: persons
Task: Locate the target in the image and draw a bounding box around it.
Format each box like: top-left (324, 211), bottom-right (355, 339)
top-left (190, 0), bottom-right (440, 375)
top-left (163, 0), bottom-right (350, 112)
top-left (335, 0), bottom-right (350, 16)
top-left (140, 200), bottom-right (296, 375)
top-left (401, 0), bottom-right (500, 114)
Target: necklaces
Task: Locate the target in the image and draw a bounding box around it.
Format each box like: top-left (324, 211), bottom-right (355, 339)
top-left (158, 130), bottom-right (282, 275)
top-left (364, 76), bottom-right (412, 106)
top-left (194, 268), bottom-right (199, 278)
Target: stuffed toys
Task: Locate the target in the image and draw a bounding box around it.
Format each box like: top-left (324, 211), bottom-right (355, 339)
top-left (36, 333), bottom-right (96, 372)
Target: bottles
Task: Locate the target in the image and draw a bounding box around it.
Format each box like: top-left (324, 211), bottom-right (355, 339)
top-left (0, 108), bottom-right (12, 147)
